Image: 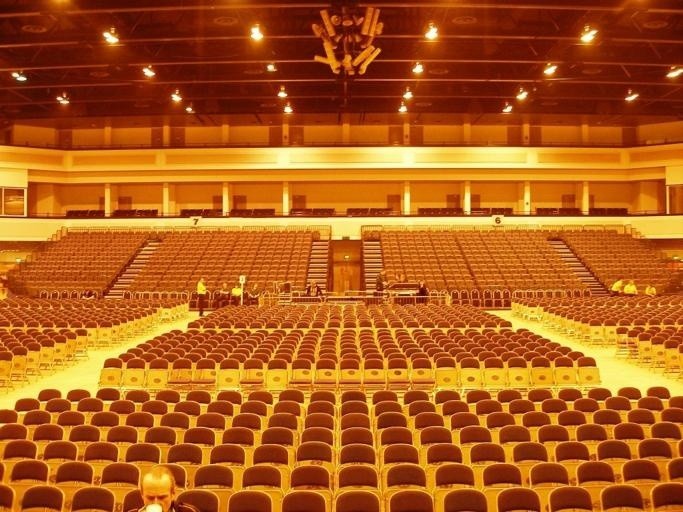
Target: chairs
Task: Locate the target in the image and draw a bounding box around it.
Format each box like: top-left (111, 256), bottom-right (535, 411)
top-left (1, 224), bottom-right (681, 291)
top-left (1, 291), bottom-right (681, 511)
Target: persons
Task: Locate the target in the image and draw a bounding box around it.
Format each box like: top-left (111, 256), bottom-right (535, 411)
top-left (307, 281), bottom-right (322, 297)
top-left (247, 283), bottom-right (261, 306)
top-left (610, 274), bottom-right (625, 297)
top-left (231, 283), bottom-right (241, 305)
top-left (624, 279), bottom-right (639, 297)
top-left (645, 283), bottom-right (657, 298)
top-left (82, 289), bottom-right (97, 299)
top-left (417, 280), bottom-right (429, 296)
top-left (215, 283), bottom-right (230, 309)
top-left (197, 277), bottom-right (207, 316)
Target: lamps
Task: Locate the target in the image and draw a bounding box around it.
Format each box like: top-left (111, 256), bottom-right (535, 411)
top-left (310, 2), bottom-right (385, 76)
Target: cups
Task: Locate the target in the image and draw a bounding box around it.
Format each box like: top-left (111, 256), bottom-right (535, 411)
top-left (146, 503), bottom-right (161, 512)
